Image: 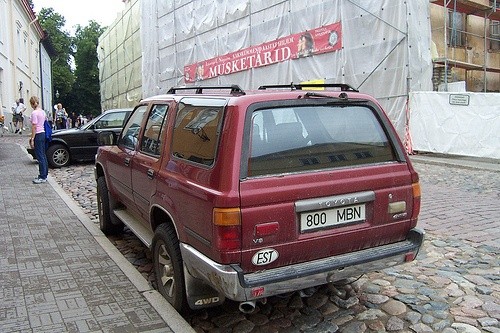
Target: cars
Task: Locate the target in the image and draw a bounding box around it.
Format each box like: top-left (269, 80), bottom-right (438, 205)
top-left (26, 108), bottom-right (136, 169)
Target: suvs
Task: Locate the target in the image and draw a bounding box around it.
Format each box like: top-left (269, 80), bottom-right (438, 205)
top-left (95, 81), bottom-right (426, 312)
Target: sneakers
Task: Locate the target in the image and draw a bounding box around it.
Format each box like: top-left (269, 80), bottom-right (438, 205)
top-left (15, 129), bottom-right (19, 134)
top-left (19, 132), bottom-right (22, 134)
top-left (33, 179), bottom-right (46, 184)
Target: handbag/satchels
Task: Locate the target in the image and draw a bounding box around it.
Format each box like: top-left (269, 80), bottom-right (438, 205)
top-left (44, 120), bottom-right (52, 142)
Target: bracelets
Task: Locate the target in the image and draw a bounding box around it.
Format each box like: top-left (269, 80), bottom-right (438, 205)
top-left (31, 137), bottom-right (34, 140)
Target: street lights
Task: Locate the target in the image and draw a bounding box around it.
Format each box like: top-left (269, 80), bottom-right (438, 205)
top-left (52, 90), bottom-right (60, 120)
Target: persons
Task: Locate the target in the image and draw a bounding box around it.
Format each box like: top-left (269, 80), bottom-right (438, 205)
top-left (293, 32), bottom-right (314, 57)
top-left (29, 96), bottom-right (52, 183)
top-left (0, 98), bottom-right (26, 135)
top-left (195, 62), bottom-right (204, 81)
top-left (48, 103), bottom-right (94, 130)
